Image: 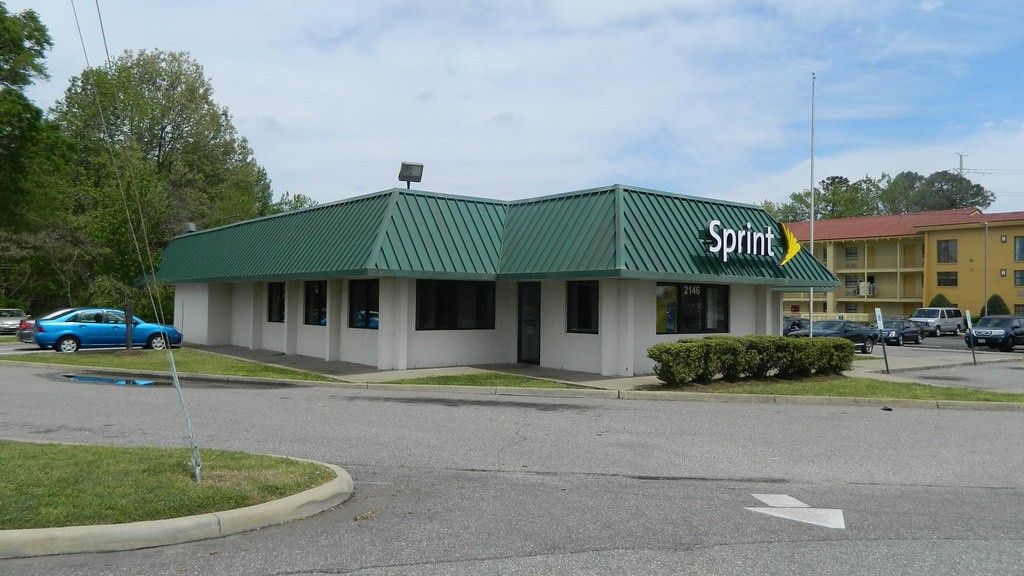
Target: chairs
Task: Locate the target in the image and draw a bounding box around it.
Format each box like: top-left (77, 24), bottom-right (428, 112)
top-left (95, 314), bottom-right (103, 323)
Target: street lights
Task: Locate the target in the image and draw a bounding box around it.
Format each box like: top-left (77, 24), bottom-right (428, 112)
top-left (809, 70), bottom-right (815, 337)
top-left (979, 219), bottom-right (988, 317)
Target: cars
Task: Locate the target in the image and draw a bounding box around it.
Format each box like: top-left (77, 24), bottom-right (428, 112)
top-left (16, 307), bottom-right (126, 350)
top-left (33, 307), bottom-right (184, 353)
top-left (965, 315), bottom-right (1024, 353)
top-left (353, 310), bottom-right (379, 329)
top-left (788, 320), bottom-right (879, 354)
top-left (782, 317), bottom-right (814, 337)
top-left (0, 308), bottom-right (32, 335)
top-left (871, 318), bottom-right (922, 346)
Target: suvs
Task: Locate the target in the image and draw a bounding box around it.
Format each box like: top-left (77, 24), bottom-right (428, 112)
top-left (908, 307), bottom-right (964, 337)
